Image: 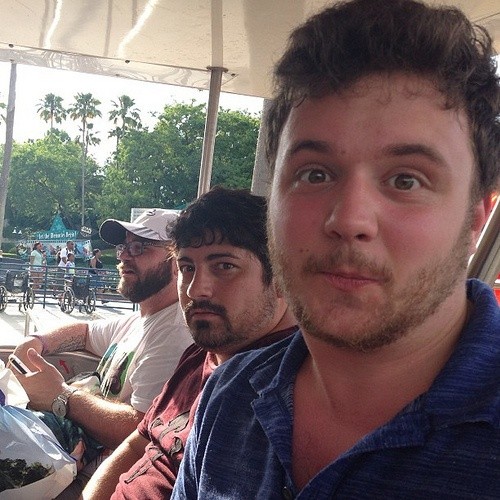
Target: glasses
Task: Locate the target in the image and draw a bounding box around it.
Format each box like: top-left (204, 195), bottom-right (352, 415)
top-left (114, 240), bottom-right (169, 258)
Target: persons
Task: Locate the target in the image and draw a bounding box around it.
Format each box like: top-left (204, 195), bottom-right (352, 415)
top-left (1, 1), bottom-right (499, 499)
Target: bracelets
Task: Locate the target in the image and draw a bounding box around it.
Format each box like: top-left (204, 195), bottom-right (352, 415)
top-left (26, 333), bottom-right (48, 356)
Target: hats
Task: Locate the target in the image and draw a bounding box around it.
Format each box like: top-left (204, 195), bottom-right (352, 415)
top-left (98, 208), bottom-right (184, 247)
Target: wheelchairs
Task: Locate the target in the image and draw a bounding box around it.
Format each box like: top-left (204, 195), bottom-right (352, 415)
top-left (0, 270), bottom-right (36, 313)
top-left (52, 274), bottom-right (98, 315)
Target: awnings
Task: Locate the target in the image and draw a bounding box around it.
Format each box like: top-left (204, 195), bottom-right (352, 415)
top-left (26, 213), bottom-right (93, 270)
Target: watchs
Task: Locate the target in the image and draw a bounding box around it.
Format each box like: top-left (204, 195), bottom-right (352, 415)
top-left (50, 386), bottom-right (80, 424)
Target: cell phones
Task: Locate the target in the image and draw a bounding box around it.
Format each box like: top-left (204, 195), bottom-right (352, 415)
top-left (9, 354), bottom-right (32, 375)
